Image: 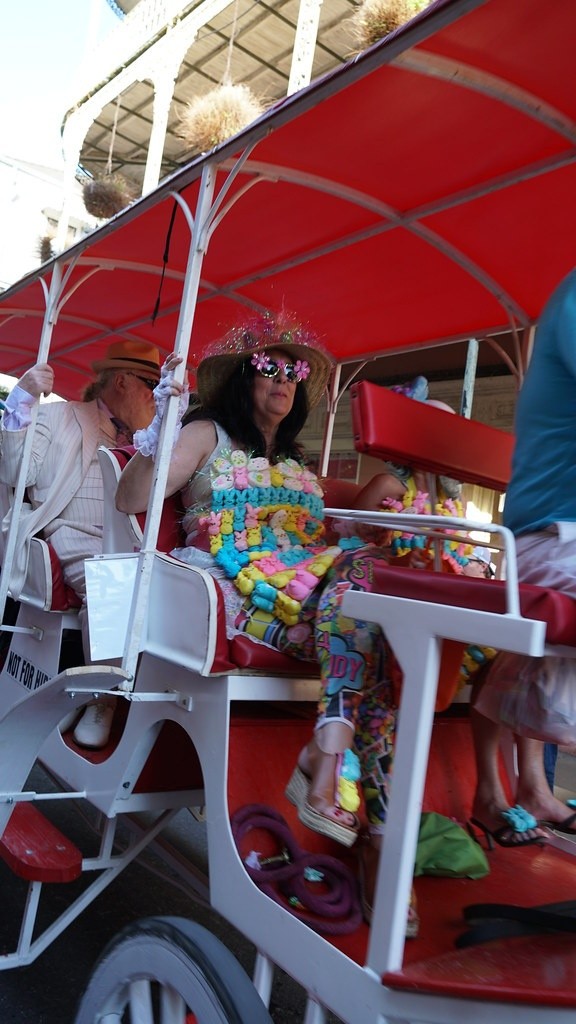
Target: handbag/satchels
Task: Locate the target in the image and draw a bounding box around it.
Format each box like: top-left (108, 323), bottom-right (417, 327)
top-left (413, 811), bottom-right (491, 880)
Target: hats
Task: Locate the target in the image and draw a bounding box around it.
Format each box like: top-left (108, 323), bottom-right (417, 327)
top-left (196, 312), bottom-right (331, 413)
top-left (390, 375), bottom-right (429, 479)
top-left (91, 340), bottom-right (161, 375)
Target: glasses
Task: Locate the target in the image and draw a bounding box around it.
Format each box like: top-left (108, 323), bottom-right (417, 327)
top-left (252, 350), bottom-right (311, 383)
top-left (126, 371), bottom-right (160, 391)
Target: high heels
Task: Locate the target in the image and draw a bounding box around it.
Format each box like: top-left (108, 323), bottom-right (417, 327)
top-left (356, 825), bottom-right (419, 937)
top-left (469, 804), bottom-right (548, 849)
top-left (284, 736), bottom-right (361, 848)
top-left (536, 799), bottom-right (576, 835)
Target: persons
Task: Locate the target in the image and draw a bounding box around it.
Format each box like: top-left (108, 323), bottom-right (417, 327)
top-left (0, 338), bottom-right (163, 747)
top-left (115, 323), bottom-right (419, 935)
top-left (349, 400), bottom-right (576, 847)
top-left (503, 267), bottom-right (576, 592)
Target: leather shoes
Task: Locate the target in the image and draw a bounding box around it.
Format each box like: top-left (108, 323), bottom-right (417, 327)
top-left (73, 702), bottom-right (117, 749)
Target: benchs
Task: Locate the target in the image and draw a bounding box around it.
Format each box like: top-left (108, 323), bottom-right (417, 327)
top-left (322, 377), bottom-right (576, 975)
top-left (6, 486), bottom-right (83, 615)
top-left (83, 446), bottom-right (366, 703)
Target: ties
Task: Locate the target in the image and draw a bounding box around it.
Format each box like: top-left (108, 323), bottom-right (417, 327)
top-left (110, 417), bottom-right (133, 448)
